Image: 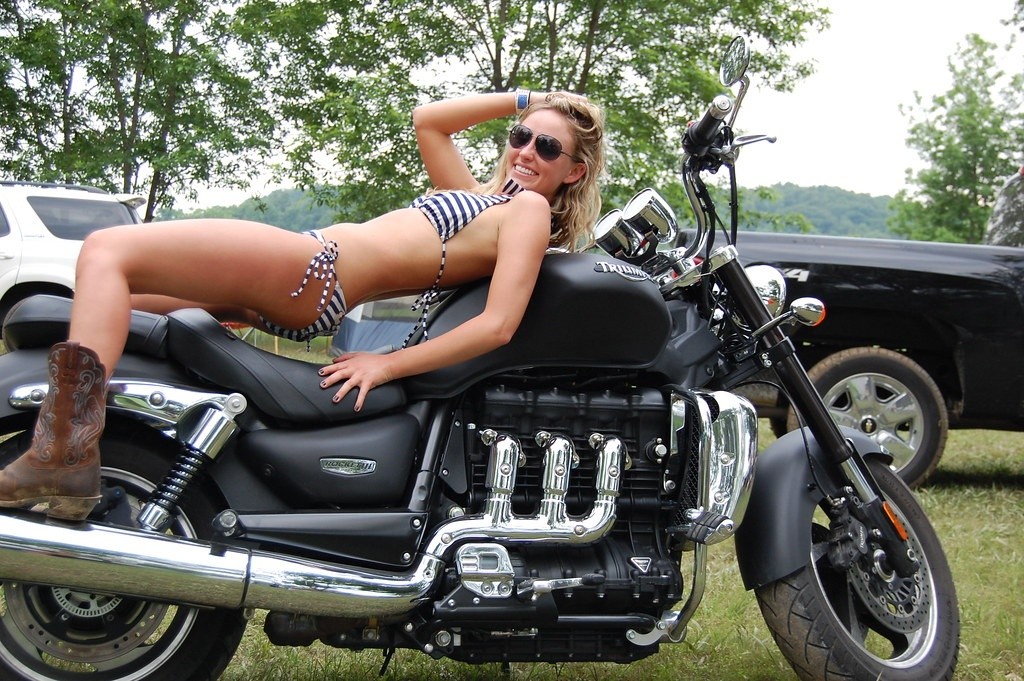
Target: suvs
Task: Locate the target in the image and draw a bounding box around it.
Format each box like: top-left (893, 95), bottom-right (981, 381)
top-left (0, 180), bottom-right (147, 349)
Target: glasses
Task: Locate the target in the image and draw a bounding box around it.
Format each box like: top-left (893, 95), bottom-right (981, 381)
top-left (509, 125), bottom-right (579, 163)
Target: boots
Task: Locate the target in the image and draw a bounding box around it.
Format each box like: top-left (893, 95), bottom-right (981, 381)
top-left (0, 340), bottom-right (114, 520)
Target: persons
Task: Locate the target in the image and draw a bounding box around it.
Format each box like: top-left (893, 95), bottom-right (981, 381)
top-left (0, 87), bottom-right (605, 524)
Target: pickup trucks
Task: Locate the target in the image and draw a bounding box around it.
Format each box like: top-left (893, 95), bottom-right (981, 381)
top-left (675, 167), bottom-right (1024, 490)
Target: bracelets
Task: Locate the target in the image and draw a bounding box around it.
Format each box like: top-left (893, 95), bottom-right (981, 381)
top-left (515, 88), bottom-right (531, 114)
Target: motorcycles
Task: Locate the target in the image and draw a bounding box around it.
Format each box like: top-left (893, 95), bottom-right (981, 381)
top-left (1, 37), bottom-right (960, 681)
